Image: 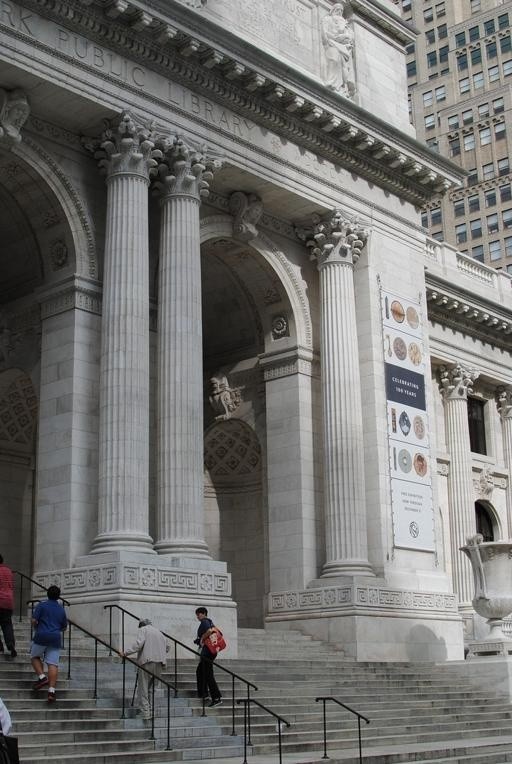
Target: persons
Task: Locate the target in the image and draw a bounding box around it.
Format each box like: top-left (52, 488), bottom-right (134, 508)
top-left (321, 2), bottom-right (356, 95)
top-left (0, 552), bottom-right (18, 656)
top-left (0, 695), bottom-right (19, 763)
top-left (194, 607), bottom-right (223, 707)
top-left (119, 619), bottom-right (167, 719)
top-left (29, 585), bottom-right (68, 703)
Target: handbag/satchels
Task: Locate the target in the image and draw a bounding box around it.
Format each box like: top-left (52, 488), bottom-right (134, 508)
top-left (201, 628), bottom-right (226, 655)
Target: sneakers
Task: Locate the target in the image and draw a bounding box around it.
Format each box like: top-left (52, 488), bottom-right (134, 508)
top-left (32, 677), bottom-right (48, 690)
top-left (205, 697), bottom-right (222, 708)
top-left (48, 692), bottom-right (56, 702)
